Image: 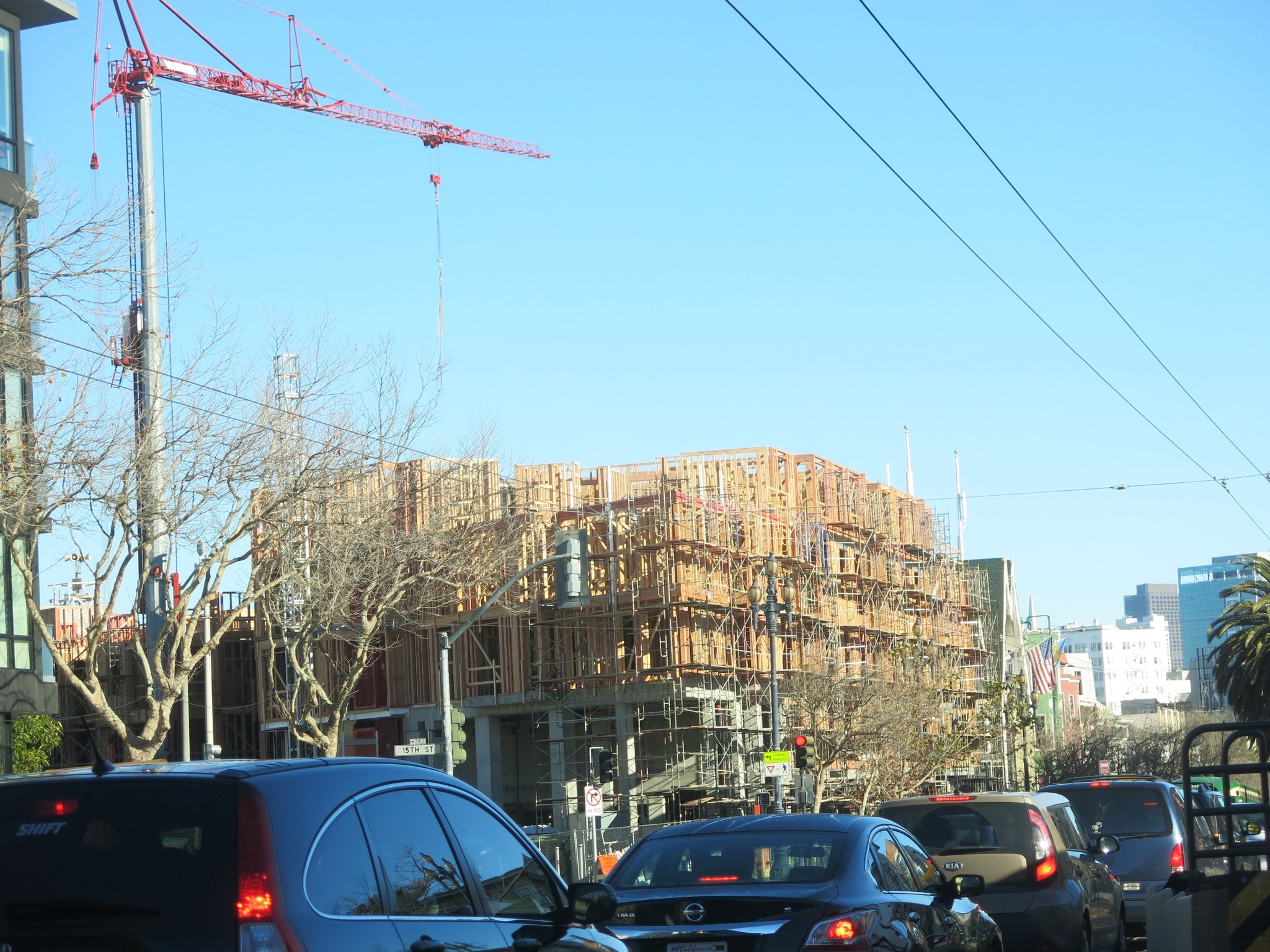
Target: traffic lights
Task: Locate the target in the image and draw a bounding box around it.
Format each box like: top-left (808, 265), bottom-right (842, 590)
top-left (599, 748), bottom-right (613, 784)
top-left (794, 736), bottom-right (815, 768)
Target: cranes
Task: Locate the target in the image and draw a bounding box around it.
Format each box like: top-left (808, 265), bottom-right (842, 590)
top-left (82, 1), bottom-right (551, 765)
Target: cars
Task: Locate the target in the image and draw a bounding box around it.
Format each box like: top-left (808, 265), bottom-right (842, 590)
top-left (587, 813), bottom-right (1005, 952)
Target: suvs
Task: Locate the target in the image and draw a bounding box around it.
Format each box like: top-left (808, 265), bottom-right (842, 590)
top-left (0, 744), bottom-right (638, 951)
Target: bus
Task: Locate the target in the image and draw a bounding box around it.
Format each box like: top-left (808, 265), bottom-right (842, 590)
top-left (1170, 775), bottom-right (1265, 834)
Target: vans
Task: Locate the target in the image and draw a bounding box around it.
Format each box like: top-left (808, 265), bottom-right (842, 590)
top-left (1035, 771), bottom-right (1262, 941)
top-left (867, 790), bottom-right (1132, 952)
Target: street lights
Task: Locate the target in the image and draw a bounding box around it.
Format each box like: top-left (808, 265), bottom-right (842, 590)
top-left (1015, 667), bottom-right (1040, 793)
top-left (746, 550), bottom-right (796, 814)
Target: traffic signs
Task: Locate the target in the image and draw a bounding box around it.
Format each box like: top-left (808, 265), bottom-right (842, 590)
top-left (763, 750), bottom-right (790, 778)
